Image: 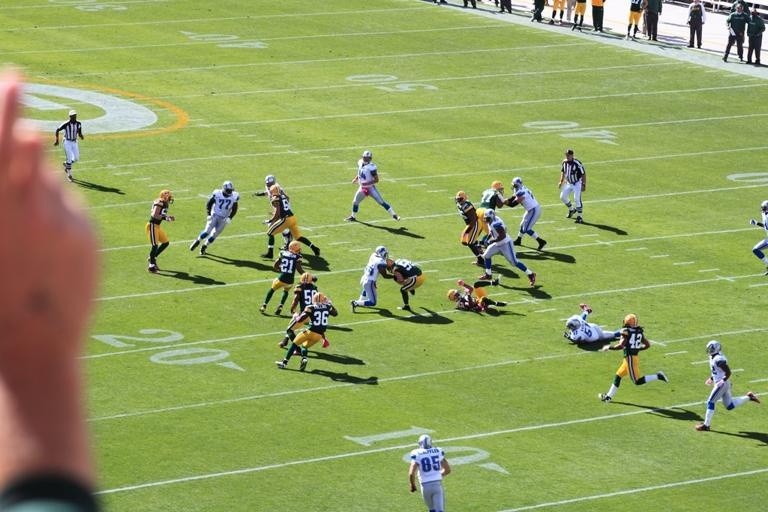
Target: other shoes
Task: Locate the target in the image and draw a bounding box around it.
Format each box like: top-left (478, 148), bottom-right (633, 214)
top-left (687, 44), bottom-right (694, 48)
top-left (648, 37), bottom-right (651, 40)
top-left (633, 35), bottom-right (636, 38)
top-left (499, 8), bottom-right (505, 13)
top-left (627, 33), bottom-right (630, 38)
top-left (509, 10), bottom-right (512, 14)
top-left (560, 21), bottom-right (562, 25)
top-left (755, 60), bottom-right (760, 64)
top-left (465, 5), bottom-right (467, 8)
top-left (549, 20), bottom-right (554, 24)
top-left (698, 45), bottom-right (700, 48)
top-left (746, 60), bottom-right (751, 64)
top-left (594, 27), bottom-right (601, 32)
top-left (531, 13), bottom-right (536, 21)
top-left (653, 38), bottom-right (657, 41)
top-left (571, 24), bottom-right (577, 31)
top-left (722, 58), bottom-right (727, 62)
top-left (579, 27), bottom-right (582, 31)
top-left (473, 6), bottom-right (476, 8)
top-left (739, 58), bottom-right (743, 63)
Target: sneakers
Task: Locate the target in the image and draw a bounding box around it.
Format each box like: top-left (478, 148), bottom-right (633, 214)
top-left (598, 392), bottom-right (612, 403)
top-left (258, 244), bottom-right (289, 315)
top-left (344, 215), bottom-right (356, 221)
top-left (392, 213), bottom-right (401, 221)
top-left (580, 303), bottom-right (592, 313)
top-left (472, 208), bottom-right (584, 307)
top-left (746, 391), bottom-right (760, 404)
top-left (274, 338), bottom-right (330, 372)
top-left (313, 247), bottom-right (321, 258)
top-left (656, 371), bottom-right (670, 385)
top-left (146, 257), bottom-right (160, 274)
top-left (350, 299), bottom-right (358, 313)
top-left (190, 242), bottom-right (205, 255)
top-left (62, 161), bottom-right (73, 182)
top-left (695, 423), bottom-right (710, 431)
top-left (396, 304), bottom-right (411, 311)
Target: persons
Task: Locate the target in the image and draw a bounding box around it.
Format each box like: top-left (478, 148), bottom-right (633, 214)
top-left (344, 150), bottom-right (402, 222)
top-left (143, 190), bottom-right (176, 273)
top-left (351, 245), bottom-right (423, 314)
top-left (622, 0), bottom-right (768, 65)
top-left (696, 340), bottom-right (761, 430)
top-left (189, 181), bottom-right (239, 255)
top-left (0, 63), bottom-right (106, 511)
top-left (448, 177), bottom-right (547, 311)
top-left (433, 0), bottom-right (608, 36)
top-left (598, 313), bottom-right (669, 404)
top-left (408, 434), bottom-right (451, 512)
top-left (562, 303), bottom-right (625, 346)
top-left (52, 110), bottom-right (85, 182)
top-left (748, 201), bottom-right (768, 275)
top-left (253, 174), bottom-right (338, 371)
top-left (558, 148), bottom-right (587, 222)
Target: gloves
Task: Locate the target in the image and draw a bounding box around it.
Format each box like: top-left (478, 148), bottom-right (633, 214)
top-left (750, 218), bottom-right (758, 226)
top-left (312, 275), bottom-right (318, 282)
top-left (705, 377), bottom-right (714, 386)
top-left (166, 215), bottom-right (175, 222)
top-left (457, 280), bottom-right (464, 286)
top-left (207, 216), bottom-right (211, 220)
top-left (715, 379), bottom-right (725, 388)
top-left (224, 217), bottom-right (232, 224)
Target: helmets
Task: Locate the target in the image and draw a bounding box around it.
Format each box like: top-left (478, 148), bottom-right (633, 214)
top-left (300, 272), bottom-right (312, 284)
top-left (159, 189), bottom-right (173, 202)
top-left (704, 339), bottom-right (721, 355)
top-left (362, 150), bottom-right (373, 160)
top-left (222, 181), bottom-right (234, 193)
top-left (311, 292), bottom-right (326, 304)
top-left (289, 241), bottom-right (300, 254)
top-left (456, 176), bottom-right (523, 223)
top-left (375, 245), bottom-right (396, 270)
top-left (447, 289), bottom-right (458, 302)
top-left (565, 317), bottom-right (581, 330)
top-left (265, 175), bottom-right (275, 186)
top-left (417, 434), bottom-right (432, 449)
top-left (761, 200), bottom-right (768, 212)
top-left (624, 314), bottom-right (638, 327)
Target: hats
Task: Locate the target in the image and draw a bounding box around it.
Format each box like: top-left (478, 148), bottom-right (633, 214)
top-left (564, 149), bottom-right (573, 155)
top-left (69, 110), bottom-right (77, 116)
